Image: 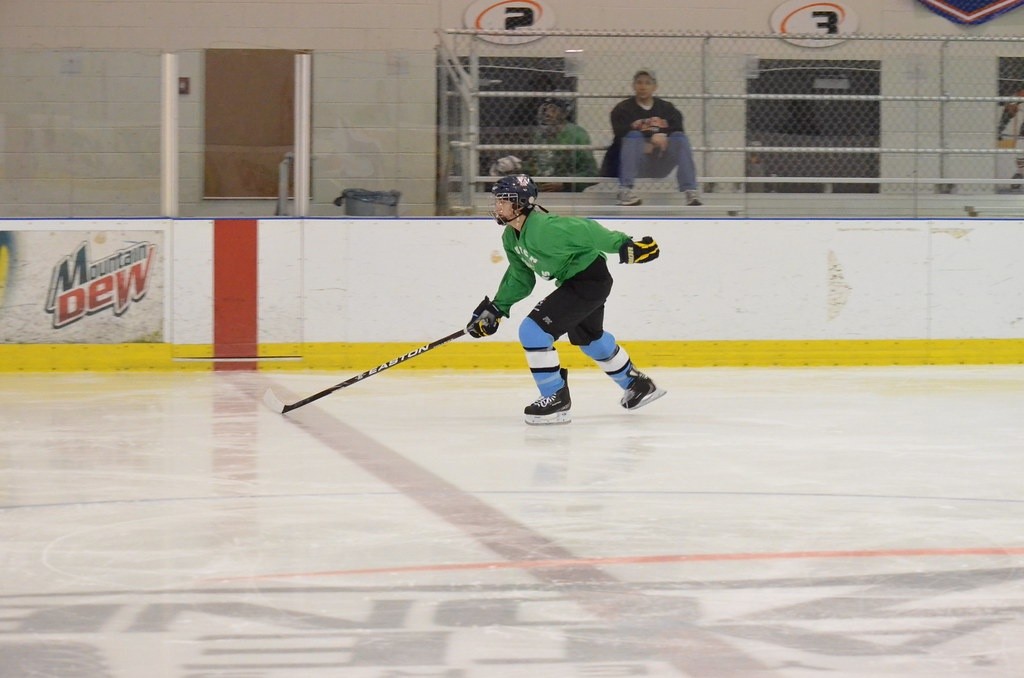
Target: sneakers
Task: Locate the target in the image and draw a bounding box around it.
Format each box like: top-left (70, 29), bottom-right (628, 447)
top-left (615, 188), bottom-right (642, 205)
top-left (523, 369), bottom-right (571, 415)
top-left (621, 367), bottom-right (656, 409)
top-left (686, 190), bottom-right (703, 205)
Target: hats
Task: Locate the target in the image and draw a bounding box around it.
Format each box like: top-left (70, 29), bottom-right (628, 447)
top-left (634, 67), bottom-right (655, 80)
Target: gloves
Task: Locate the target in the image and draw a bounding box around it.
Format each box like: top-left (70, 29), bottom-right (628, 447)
top-left (618, 236), bottom-right (658, 264)
top-left (465, 296), bottom-right (502, 338)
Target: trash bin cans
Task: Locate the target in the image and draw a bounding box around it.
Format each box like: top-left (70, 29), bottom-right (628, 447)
top-left (345, 187), bottom-right (402, 216)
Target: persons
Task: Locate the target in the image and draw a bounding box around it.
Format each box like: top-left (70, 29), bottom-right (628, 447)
top-left (467, 174), bottom-right (667, 424)
top-left (490, 90), bottom-right (599, 192)
top-left (600, 68), bottom-right (702, 205)
top-left (998, 90), bottom-right (1024, 192)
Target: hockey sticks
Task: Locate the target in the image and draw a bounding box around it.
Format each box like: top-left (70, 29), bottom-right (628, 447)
top-left (261, 317), bottom-right (492, 415)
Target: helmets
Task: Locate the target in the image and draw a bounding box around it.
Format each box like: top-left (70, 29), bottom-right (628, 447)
top-left (537, 90), bottom-right (575, 137)
top-left (492, 174), bottom-right (538, 205)
